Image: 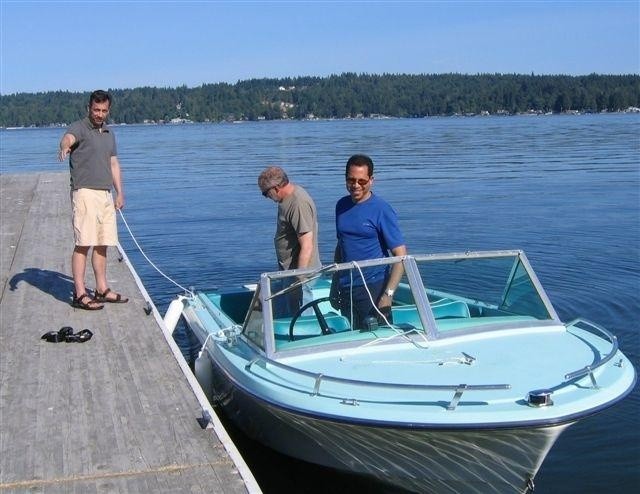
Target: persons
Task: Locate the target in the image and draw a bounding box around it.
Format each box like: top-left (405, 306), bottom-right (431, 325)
top-left (257, 164), bottom-right (324, 318)
top-left (329, 155), bottom-right (409, 331)
top-left (57, 90), bottom-right (130, 312)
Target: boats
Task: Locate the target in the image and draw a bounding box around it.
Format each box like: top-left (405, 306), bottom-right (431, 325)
top-left (178, 261), bottom-right (637, 493)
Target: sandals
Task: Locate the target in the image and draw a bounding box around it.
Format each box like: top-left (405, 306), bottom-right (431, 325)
top-left (73, 291), bottom-right (104, 309)
top-left (94, 289), bottom-right (129, 303)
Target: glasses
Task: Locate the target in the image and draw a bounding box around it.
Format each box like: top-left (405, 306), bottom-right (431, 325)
top-left (261, 184), bottom-right (279, 196)
top-left (346, 173), bottom-right (370, 186)
top-left (66, 329), bottom-right (92, 343)
top-left (42, 326), bottom-right (73, 343)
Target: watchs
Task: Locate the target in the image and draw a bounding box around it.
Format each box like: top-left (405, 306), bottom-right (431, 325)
top-left (383, 287), bottom-right (395, 298)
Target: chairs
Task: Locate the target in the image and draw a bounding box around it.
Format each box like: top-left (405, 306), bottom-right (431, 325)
top-left (273, 296), bottom-right (473, 344)
